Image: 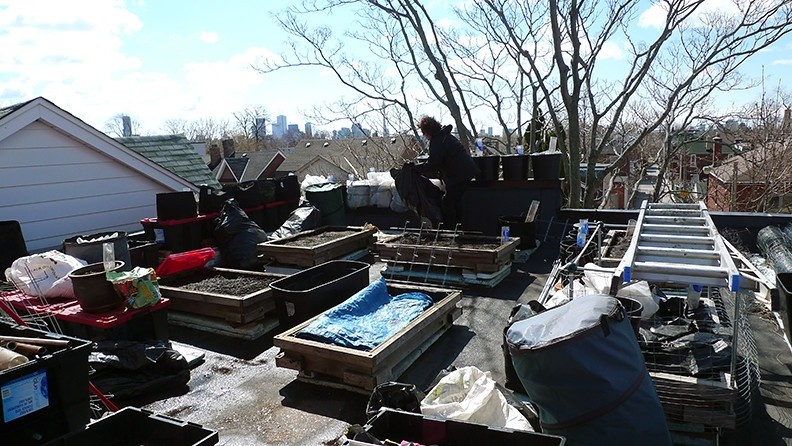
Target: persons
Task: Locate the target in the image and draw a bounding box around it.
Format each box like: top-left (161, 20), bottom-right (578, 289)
top-left (415, 115), bottom-right (481, 232)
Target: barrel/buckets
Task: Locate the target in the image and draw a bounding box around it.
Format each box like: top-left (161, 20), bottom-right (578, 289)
top-left (155, 192), bottom-right (196, 220)
top-left (560, 222), bottom-right (607, 287)
top-left (68, 260), bottom-right (127, 313)
top-left (63, 230), bottom-right (132, 273)
top-left (613, 296), bottom-right (644, 337)
top-left (471, 151), bottom-right (562, 180)
top-left (304, 183), bottom-right (347, 227)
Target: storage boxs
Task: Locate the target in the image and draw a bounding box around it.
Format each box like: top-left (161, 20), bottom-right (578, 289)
top-left (270, 258), bottom-right (371, 328)
top-left (256, 225), bottom-right (376, 268)
top-left (156, 267), bottom-right (287, 339)
top-left (377, 231), bottom-right (520, 274)
top-left (344, 405), bottom-right (567, 446)
top-left (273, 284), bottom-right (463, 395)
top-left (0, 289), bottom-right (171, 346)
top-left (594, 230), bottom-right (631, 267)
top-left (0, 320), bottom-right (93, 446)
top-left (48, 406), bottom-right (218, 446)
